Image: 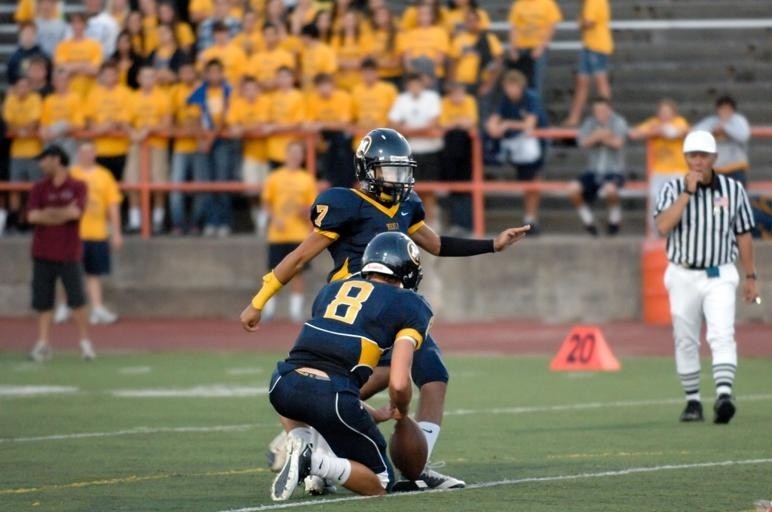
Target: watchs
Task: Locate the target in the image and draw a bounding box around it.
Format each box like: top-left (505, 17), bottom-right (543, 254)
top-left (745, 273), bottom-right (759, 281)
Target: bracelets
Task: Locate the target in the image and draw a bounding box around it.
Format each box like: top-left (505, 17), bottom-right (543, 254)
top-left (251, 267), bottom-right (284, 313)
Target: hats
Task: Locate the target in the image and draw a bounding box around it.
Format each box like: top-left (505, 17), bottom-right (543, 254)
top-left (684, 131), bottom-right (716, 154)
top-left (33, 144), bottom-right (69, 167)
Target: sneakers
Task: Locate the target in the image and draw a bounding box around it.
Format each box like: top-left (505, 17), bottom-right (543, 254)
top-left (80, 339), bottom-right (95, 360)
top-left (88, 308), bottom-right (119, 327)
top-left (29, 341), bottom-right (52, 360)
top-left (413, 461), bottom-right (465, 491)
top-left (681, 401), bottom-right (702, 421)
top-left (54, 304), bottom-right (71, 325)
top-left (304, 475), bottom-right (338, 495)
top-left (714, 399), bottom-right (735, 423)
top-left (271, 436), bottom-right (313, 502)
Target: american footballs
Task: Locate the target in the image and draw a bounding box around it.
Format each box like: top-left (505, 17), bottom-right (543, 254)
top-left (389, 416), bottom-right (427, 480)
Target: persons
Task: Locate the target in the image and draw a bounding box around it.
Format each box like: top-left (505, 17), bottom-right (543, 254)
top-left (18, 141), bottom-right (96, 362)
top-left (687, 95), bottom-right (750, 189)
top-left (3, 0), bottom-right (49, 230)
top-left (126, 0), bottom-right (265, 238)
top-left (269, 230), bottom-right (437, 502)
top-left (53, 140), bottom-right (124, 325)
top-left (258, 141), bottom-right (321, 325)
top-left (266, 0), bottom-right (306, 169)
top-left (628, 97), bottom-right (689, 238)
top-left (653, 129), bottom-right (761, 424)
top-left (315, 129), bottom-right (362, 190)
top-left (48, 0), bottom-right (126, 180)
top-left (305, 0), bottom-right (400, 152)
top-left (401, 0), bottom-right (559, 237)
top-left (566, 96), bottom-right (627, 235)
top-left (560, 0), bottom-right (615, 129)
top-left (238, 129), bottom-right (533, 490)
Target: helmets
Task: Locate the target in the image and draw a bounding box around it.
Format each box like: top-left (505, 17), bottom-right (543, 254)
top-left (355, 130), bottom-right (418, 205)
top-left (361, 232), bottom-right (423, 292)
